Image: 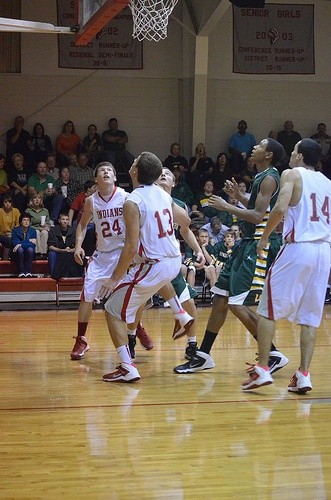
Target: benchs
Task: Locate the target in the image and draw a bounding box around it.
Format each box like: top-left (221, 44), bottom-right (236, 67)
top-left (0, 260), bottom-right (86, 294)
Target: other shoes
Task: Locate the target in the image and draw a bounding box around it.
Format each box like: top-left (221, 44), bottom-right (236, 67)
top-left (18, 273), bottom-right (32, 278)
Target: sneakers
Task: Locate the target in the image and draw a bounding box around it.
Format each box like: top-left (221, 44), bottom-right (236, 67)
top-left (173, 350), bottom-right (215, 374)
top-left (128, 334), bottom-right (137, 359)
top-left (286, 367), bottom-right (313, 392)
top-left (240, 365), bottom-right (274, 391)
top-left (135, 328), bottom-right (153, 350)
top-left (102, 364), bottom-right (141, 383)
top-left (70, 336), bottom-right (90, 360)
top-left (244, 351), bottom-right (289, 375)
top-left (172, 311), bottom-right (194, 340)
top-left (184, 341), bottom-right (199, 360)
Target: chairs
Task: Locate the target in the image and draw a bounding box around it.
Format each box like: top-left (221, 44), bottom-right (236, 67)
top-left (55, 253), bottom-right (86, 306)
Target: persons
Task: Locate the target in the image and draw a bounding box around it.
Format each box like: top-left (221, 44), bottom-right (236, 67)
top-left (1, 115), bottom-right (331, 381)
top-left (241, 140), bottom-right (331, 394)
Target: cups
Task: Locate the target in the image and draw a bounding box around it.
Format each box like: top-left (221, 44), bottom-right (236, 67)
top-left (48, 183), bottom-right (53, 188)
top-left (40, 216), bottom-right (46, 225)
top-left (61, 185), bottom-right (67, 198)
